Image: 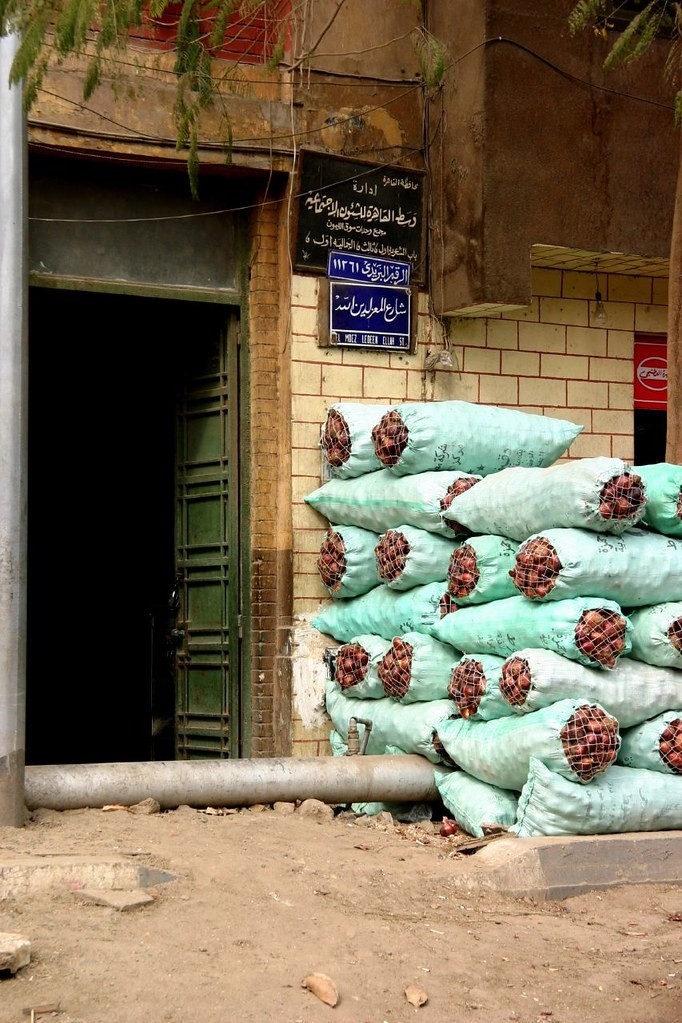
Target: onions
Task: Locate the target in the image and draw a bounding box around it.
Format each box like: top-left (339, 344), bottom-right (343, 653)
top-left (318, 409), bottom-right (682, 782)
top-left (440, 815), bottom-right (459, 837)
